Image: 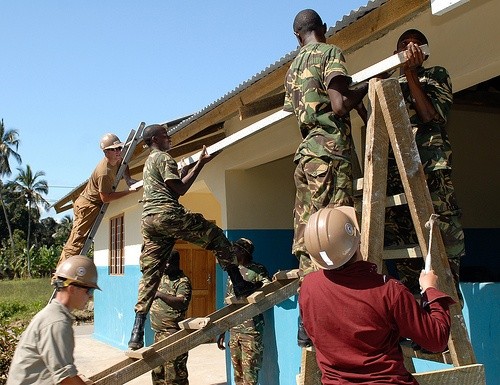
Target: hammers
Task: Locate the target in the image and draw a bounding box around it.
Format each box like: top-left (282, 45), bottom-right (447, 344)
top-left (425, 213), bottom-right (440, 270)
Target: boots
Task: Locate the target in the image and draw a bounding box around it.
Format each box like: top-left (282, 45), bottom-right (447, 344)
top-left (226, 266), bottom-right (263, 299)
top-left (128, 313), bottom-right (147, 347)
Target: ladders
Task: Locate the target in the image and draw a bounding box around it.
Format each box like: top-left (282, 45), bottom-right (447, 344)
top-left (77, 269), bottom-right (301, 384)
top-left (48, 121), bottom-right (146, 304)
top-left (301, 77), bottom-right (486, 385)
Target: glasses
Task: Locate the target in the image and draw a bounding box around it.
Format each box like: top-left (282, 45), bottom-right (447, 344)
top-left (105, 147), bottom-right (122, 151)
top-left (69, 283), bottom-right (95, 295)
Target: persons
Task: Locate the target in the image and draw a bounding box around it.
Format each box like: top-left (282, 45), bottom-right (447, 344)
top-left (4, 254), bottom-right (98, 385)
top-left (297, 203), bottom-right (454, 384)
top-left (281, 7), bottom-right (378, 350)
top-left (212, 237), bottom-right (288, 385)
top-left (126, 125), bottom-right (265, 351)
top-left (148, 249), bottom-right (193, 385)
top-left (50, 132), bottom-right (141, 286)
top-left (382, 28), bottom-right (465, 352)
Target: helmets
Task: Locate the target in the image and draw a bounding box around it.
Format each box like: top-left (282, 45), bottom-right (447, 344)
top-left (303, 206), bottom-right (361, 270)
top-left (233, 238), bottom-right (255, 256)
top-left (100, 134), bottom-right (124, 151)
top-left (52, 255), bottom-right (101, 291)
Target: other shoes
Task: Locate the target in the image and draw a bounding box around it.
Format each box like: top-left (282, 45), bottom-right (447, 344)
top-left (297, 327), bottom-right (313, 347)
